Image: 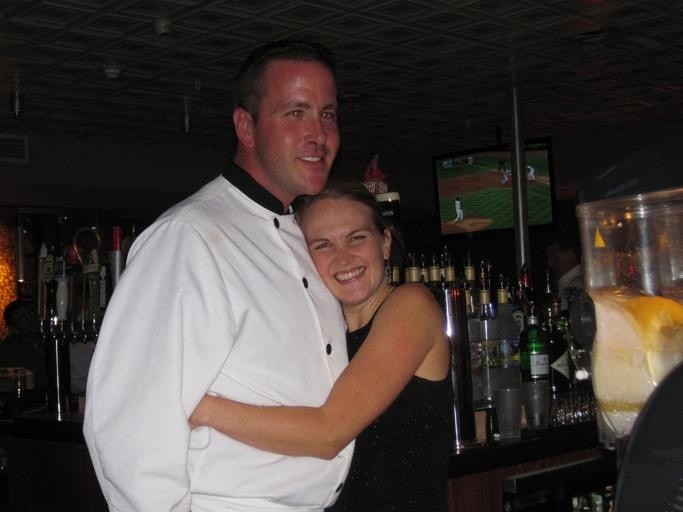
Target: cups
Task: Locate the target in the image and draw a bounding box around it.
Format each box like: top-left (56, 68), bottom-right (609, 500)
top-left (495, 379), bottom-right (551, 440)
top-left (577, 189), bottom-right (683, 449)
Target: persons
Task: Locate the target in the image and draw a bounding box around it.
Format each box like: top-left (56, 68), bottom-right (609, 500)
top-left (526, 165), bottom-right (536, 181)
top-left (496, 156), bottom-right (506, 174)
top-left (185, 176), bottom-right (455, 510)
top-left (81, 37), bottom-right (357, 512)
top-left (543, 232), bottom-right (584, 314)
top-left (440, 156), bottom-right (473, 169)
top-left (501, 166), bottom-right (511, 184)
top-left (0, 298), bottom-right (54, 386)
top-left (451, 196), bottom-right (463, 223)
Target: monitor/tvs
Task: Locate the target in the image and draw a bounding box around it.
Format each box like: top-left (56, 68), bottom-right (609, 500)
top-left (431, 135), bottom-right (559, 243)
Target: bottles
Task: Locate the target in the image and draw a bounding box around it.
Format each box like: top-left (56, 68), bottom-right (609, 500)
top-left (388, 248), bottom-right (571, 400)
